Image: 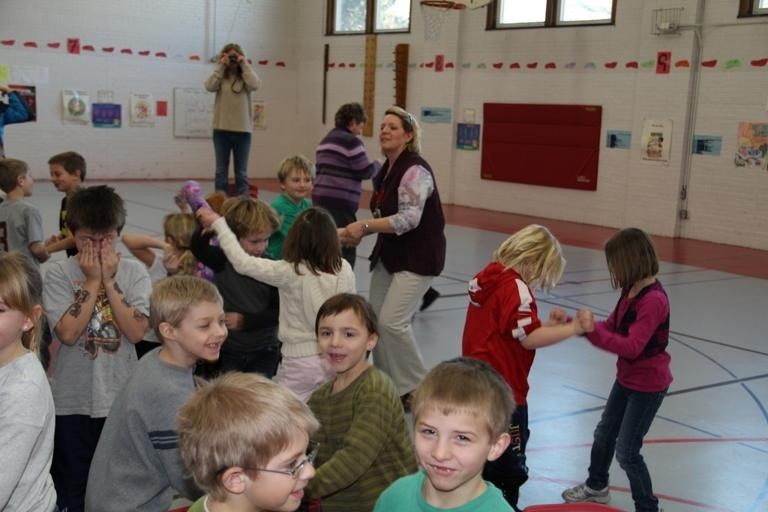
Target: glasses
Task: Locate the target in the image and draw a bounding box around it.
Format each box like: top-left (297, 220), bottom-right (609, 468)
top-left (213, 439), bottom-right (321, 479)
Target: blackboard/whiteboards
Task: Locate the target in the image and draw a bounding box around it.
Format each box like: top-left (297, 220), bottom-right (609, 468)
top-left (173, 86), bottom-right (219, 140)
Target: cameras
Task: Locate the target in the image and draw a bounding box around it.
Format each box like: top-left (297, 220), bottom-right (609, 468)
top-left (228, 55), bottom-right (237, 64)
top-left (373, 208), bottom-right (381, 218)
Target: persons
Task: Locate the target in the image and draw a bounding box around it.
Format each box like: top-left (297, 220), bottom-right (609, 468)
top-left (203, 41), bottom-right (262, 197)
top-left (560, 227), bottom-right (674, 512)
top-left (371, 354), bottom-right (520, 511)
top-left (41, 183), bottom-right (153, 512)
top-left (342, 105), bottom-right (447, 412)
top-left (84, 273), bottom-right (230, 512)
top-left (0, 250), bottom-right (59, 512)
top-left (294, 293), bottom-right (419, 511)
top-left (0, 158), bottom-right (52, 268)
top-left (0, 82), bottom-right (29, 159)
top-left (310, 102), bottom-right (384, 269)
top-left (176, 368), bottom-right (322, 512)
top-left (120, 153), bottom-right (357, 405)
top-left (461, 223), bottom-right (593, 512)
top-left (43, 151), bottom-right (88, 258)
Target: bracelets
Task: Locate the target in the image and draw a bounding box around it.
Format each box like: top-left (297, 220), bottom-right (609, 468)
top-left (361, 220), bottom-right (369, 235)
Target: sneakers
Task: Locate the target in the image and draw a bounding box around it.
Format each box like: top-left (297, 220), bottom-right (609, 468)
top-left (419, 288), bottom-right (439, 311)
top-left (562, 483), bottom-right (611, 503)
